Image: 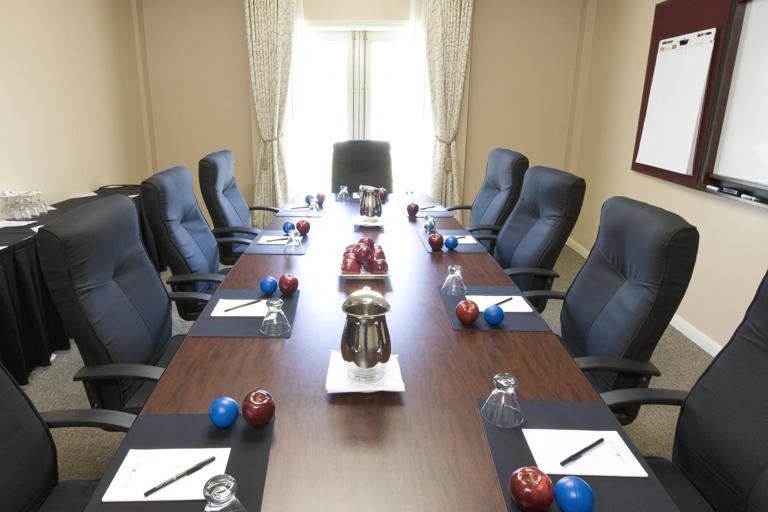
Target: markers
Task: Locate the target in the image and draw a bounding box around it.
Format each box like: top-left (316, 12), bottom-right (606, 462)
top-left (741, 193), bottom-right (760, 202)
top-left (706, 185), bottom-right (722, 192)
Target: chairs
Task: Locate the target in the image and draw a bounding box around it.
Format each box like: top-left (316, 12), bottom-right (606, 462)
top-left (472, 166), bottom-right (586, 313)
top-left (331, 139), bottom-right (393, 194)
top-left (37, 194), bottom-right (212, 432)
top-left (0, 360), bottom-right (138, 511)
top-left (599, 270), bottom-right (768, 511)
top-left (139, 165), bottom-right (234, 321)
top-left (198, 149), bottom-right (280, 265)
top-left (522, 196), bottom-right (700, 423)
top-left (444, 148), bottom-right (529, 253)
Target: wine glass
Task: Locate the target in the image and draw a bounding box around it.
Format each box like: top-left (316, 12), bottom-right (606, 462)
top-left (1, 188), bottom-right (48, 220)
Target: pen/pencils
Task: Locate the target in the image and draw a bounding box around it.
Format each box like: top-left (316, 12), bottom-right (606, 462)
top-left (496, 297), bottom-right (512, 305)
top-left (267, 238), bottom-right (288, 242)
top-left (224, 299), bottom-right (261, 312)
top-left (420, 206), bottom-right (436, 210)
top-left (144, 456), bottom-right (215, 497)
top-left (560, 438), bottom-right (605, 466)
top-left (456, 235), bottom-right (465, 239)
top-left (291, 207), bottom-right (309, 209)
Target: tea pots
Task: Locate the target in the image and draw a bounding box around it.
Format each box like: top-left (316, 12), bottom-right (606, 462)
top-left (339, 286), bottom-right (394, 382)
top-left (359, 184), bottom-right (385, 222)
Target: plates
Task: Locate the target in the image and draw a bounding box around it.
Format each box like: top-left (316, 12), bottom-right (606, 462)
top-left (351, 213), bottom-right (385, 230)
top-left (337, 260), bottom-right (390, 280)
top-left (323, 348), bottom-right (406, 396)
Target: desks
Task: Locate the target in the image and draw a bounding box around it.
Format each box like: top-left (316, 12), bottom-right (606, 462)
top-left (0, 184), bottom-right (169, 384)
top-left (84, 193), bottom-right (678, 512)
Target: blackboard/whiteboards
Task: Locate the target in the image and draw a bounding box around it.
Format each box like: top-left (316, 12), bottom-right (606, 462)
top-left (709, 0), bottom-right (768, 189)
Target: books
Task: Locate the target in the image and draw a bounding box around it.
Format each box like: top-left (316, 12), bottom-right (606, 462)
top-left (257, 235), bottom-right (303, 246)
top-left (521, 428), bottom-right (649, 479)
top-left (419, 205), bottom-right (445, 211)
top-left (102, 447), bottom-right (232, 503)
top-left (443, 235), bottom-right (477, 245)
top-left (465, 295), bottom-right (533, 313)
top-left (211, 299), bottom-right (269, 317)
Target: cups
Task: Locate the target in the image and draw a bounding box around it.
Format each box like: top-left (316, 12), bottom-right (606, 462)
top-left (283, 229), bottom-right (304, 254)
top-left (200, 473), bottom-right (247, 510)
top-left (306, 199), bottom-right (320, 216)
top-left (479, 372), bottom-right (527, 428)
top-left (335, 185), bottom-right (350, 201)
top-left (439, 264), bottom-right (470, 296)
top-left (258, 297), bottom-right (292, 338)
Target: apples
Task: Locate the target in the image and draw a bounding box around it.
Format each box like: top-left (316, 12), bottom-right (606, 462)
top-left (278, 273), bottom-right (299, 296)
top-left (242, 390), bottom-right (283, 429)
top-left (380, 187), bottom-right (388, 199)
top-left (429, 233), bottom-right (443, 250)
top-left (509, 466), bottom-right (555, 511)
top-left (316, 192), bottom-right (326, 205)
top-left (407, 202), bottom-right (419, 215)
top-left (295, 220), bottom-right (311, 234)
top-left (340, 238), bottom-right (389, 274)
top-left (456, 300), bottom-right (479, 325)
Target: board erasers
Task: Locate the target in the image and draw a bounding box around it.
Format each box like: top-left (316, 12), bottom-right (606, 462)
top-left (722, 187), bottom-right (740, 196)
